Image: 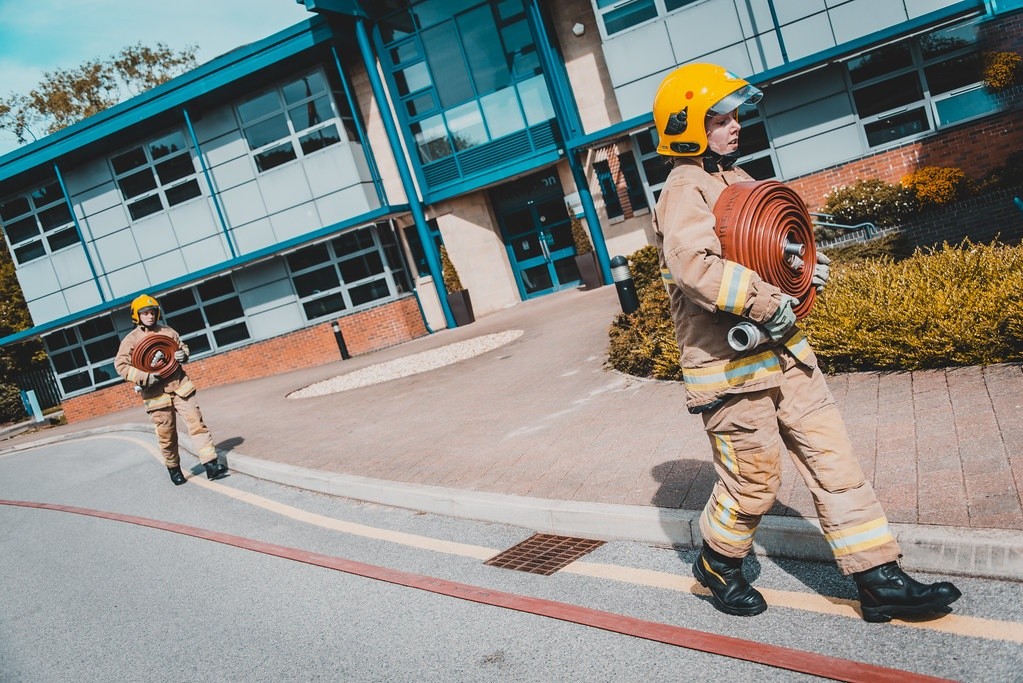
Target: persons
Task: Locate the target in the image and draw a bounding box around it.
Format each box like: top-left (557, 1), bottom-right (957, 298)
top-left (651, 61), bottom-right (963, 623)
top-left (114, 293), bottom-right (229, 484)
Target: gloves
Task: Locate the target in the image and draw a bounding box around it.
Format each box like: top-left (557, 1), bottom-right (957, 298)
top-left (762, 293), bottom-right (800, 343)
top-left (174, 350), bottom-right (187, 363)
top-left (146, 373), bottom-right (161, 387)
top-left (812, 251), bottom-right (832, 295)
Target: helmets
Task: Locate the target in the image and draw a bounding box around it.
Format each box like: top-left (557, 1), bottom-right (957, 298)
top-left (652, 63), bottom-right (763, 157)
top-left (129, 294), bottom-right (161, 324)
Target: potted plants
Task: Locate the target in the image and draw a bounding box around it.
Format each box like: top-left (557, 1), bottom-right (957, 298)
top-left (441, 244), bottom-right (475, 326)
top-left (566, 201), bottom-right (602, 290)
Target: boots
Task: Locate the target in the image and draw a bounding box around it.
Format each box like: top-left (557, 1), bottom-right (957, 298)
top-left (166, 465), bottom-right (187, 485)
top-left (691, 538), bottom-right (768, 617)
top-left (852, 560), bottom-right (962, 623)
top-left (202, 459), bottom-right (226, 481)
